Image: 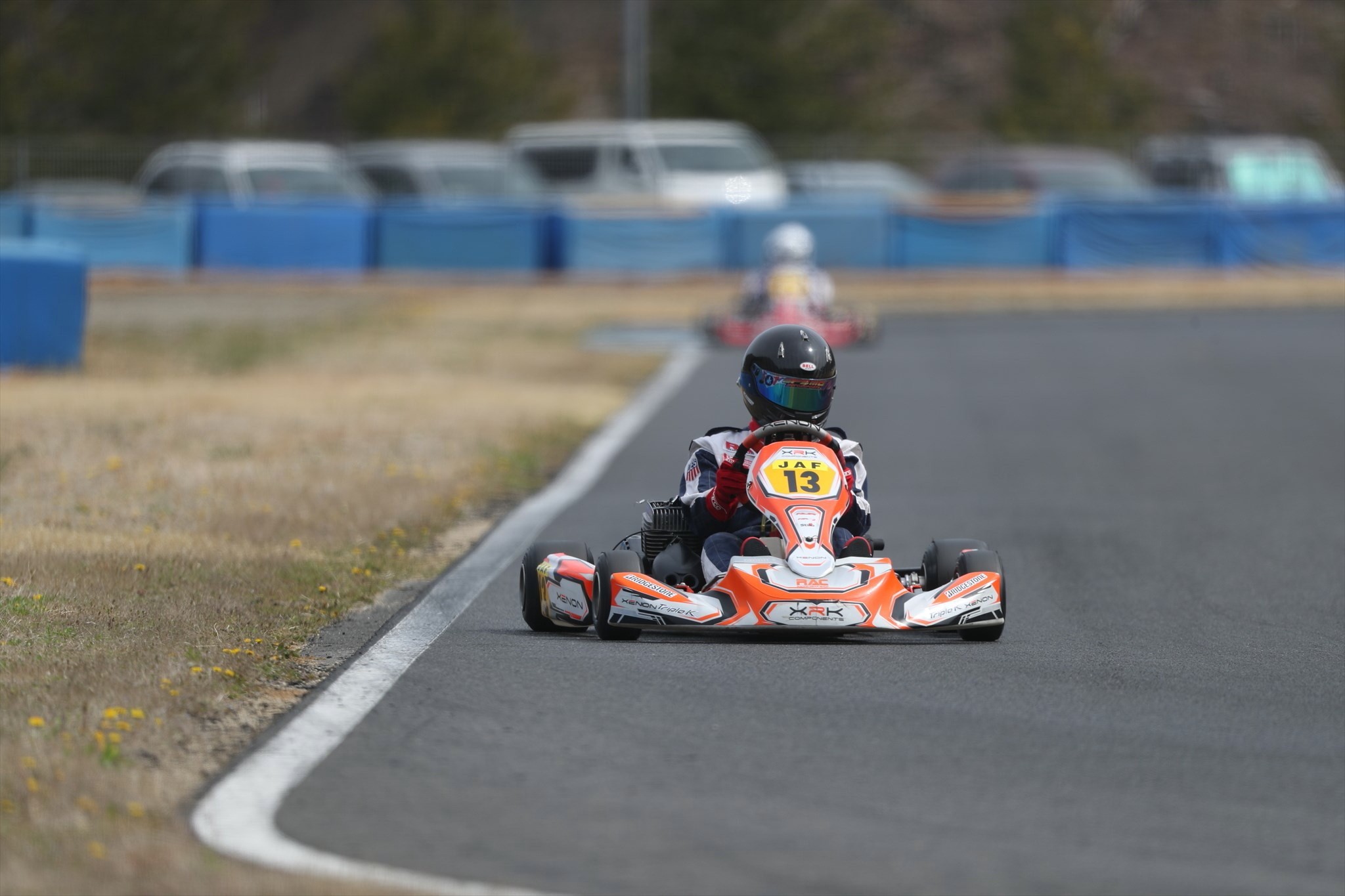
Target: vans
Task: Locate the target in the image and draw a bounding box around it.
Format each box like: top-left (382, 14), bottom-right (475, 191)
top-left (506, 117), bottom-right (793, 213)
top-left (1138, 132), bottom-right (1345, 201)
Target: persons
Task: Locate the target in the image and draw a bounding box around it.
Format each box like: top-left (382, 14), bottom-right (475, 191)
top-left (679, 331), bottom-right (872, 589)
top-left (738, 225), bottom-right (835, 320)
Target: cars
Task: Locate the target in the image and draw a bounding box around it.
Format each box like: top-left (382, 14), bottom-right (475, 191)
top-left (786, 156), bottom-right (930, 208)
top-left (347, 139), bottom-right (534, 197)
top-left (918, 148), bottom-right (1138, 204)
top-left (0, 174), bottom-right (138, 207)
top-left (135, 138), bottom-right (371, 204)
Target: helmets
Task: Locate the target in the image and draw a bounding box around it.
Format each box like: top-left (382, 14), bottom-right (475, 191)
top-left (761, 223), bottom-right (815, 263)
top-left (735, 323), bottom-right (838, 429)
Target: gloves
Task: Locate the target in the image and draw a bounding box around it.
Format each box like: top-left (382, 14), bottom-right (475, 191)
top-left (706, 458), bottom-right (749, 522)
top-left (843, 467), bottom-right (855, 516)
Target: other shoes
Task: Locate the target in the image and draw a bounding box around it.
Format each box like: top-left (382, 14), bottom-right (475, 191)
top-left (839, 536), bottom-right (874, 558)
top-left (741, 537), bottom-right (772, 557)
